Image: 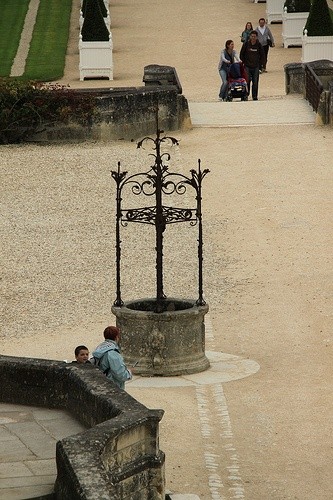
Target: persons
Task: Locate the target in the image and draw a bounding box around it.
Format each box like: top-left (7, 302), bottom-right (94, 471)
top-left (239, 30), bottom-right (265, 101)
top-left (70, 345), bottom-right (92, 364)
top-left (218, 39), bottom-right (243, 102)
top-left (240, 22), bottom-right (254, 42)
top-left (254, 17), bottom-right (276, 73)
top-left (91, 325), bottom-right (135, 393)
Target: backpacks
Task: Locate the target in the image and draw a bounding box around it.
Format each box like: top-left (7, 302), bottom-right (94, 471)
top-left (88, 357), bottom-right (110, 377)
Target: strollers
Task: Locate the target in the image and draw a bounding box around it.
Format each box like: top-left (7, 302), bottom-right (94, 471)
top-left (225, 62), bottom-right (249, 102)
